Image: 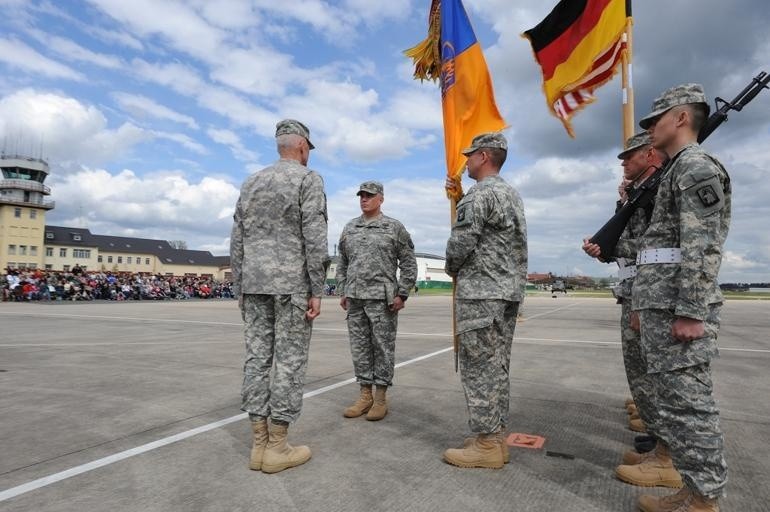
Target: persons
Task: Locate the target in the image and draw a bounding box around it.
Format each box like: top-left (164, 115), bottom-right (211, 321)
top-left (228, 119), bottom-right (332, 474)
top-left (442, 132), bottom-right (529, 470)
top-left (334, 180), bottom-right (418, 423)
top-left (632, 83), bottom-right (732, 510)
top-left (581, 128), bottom-right (684, 489)
top-left (5, 262), bottom-right (235, 301)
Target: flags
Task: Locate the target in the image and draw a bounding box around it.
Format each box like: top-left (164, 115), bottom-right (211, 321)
top-left (402, 0), bottom-right (512, 197)
top-left (519, 0), bottom-right (633, 140)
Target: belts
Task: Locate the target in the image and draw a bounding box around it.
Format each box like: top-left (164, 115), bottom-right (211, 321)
top-left (622, 265), bottom-right (637, 280)
top-left (636, 248), bottom-right (683, 269)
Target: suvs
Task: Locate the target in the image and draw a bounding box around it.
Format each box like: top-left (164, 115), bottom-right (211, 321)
top-left (551, 279), bottom-right (567, 294)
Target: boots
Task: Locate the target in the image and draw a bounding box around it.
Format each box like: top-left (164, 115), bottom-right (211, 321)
top-left (261, 422), bottom-right (312, 475)
top-left (248, 421), bottom-right (268, 472)
top-left (463, 436), bottom-right (510, 466)
top-left (616, 395), bottom-right (684, 491)
top-left (365, 385), bottom-right (389, 422)
top-left (443, 436), bottom-right (505, 471)
top-left (342, 384), bottom-right (374, 419)
top-left (639, 481), bottom-right (721, 511)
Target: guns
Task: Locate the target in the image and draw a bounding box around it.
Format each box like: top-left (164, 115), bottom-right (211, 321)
top-left (589, 71), bottom-right (770, 257)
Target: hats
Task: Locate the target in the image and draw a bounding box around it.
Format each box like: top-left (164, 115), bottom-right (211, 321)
top-left (275, 119), bottom-right (316, 150)
top-left (640, 82), bottom-right (708, 131)
top-left (616, 128), bottom-right (660, 159)
top-left (356, 180), bottom-right (384, 197)
top-left (460, 131), bottom-right (509, 157)
